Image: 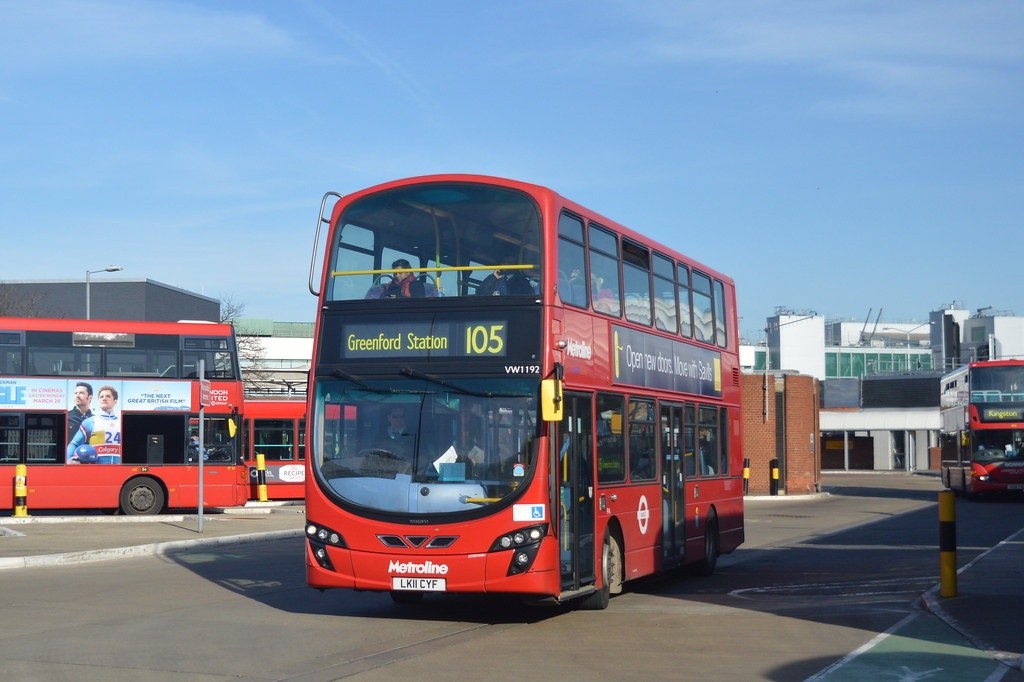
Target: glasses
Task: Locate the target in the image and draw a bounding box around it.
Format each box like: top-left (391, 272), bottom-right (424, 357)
top-left (73, 459), bottom-right (97, 464)
top-left (390, 413), bottom-right (406, 420)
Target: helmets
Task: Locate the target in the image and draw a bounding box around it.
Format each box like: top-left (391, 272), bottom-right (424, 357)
top-left (74, 444), bottom-right (98, 461)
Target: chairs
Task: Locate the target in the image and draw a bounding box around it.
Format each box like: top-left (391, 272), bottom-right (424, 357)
top-left (569, 276), bottom-right (586, 307)
top-left (558, 269), bottom-right (571, 303)
top-left (365, 275), bottom-right (394, 300)
top-left (416, 273), bottom-right (439, 298)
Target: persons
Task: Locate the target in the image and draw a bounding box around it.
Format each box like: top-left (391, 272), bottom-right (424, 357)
top-left (189, 431), bottom-right (230, 461)
top-left (476, 253), bottom-right (618, 315)
top-left (452, 419), bottom-right (715, 480)
top-left (68, 382), bottom-right (121, 464)
top-left (384, 260), bottom-right (426, 298)
top-left (370, 403), bottom-right (415, 461)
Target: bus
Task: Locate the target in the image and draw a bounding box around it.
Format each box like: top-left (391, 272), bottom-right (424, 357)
top-left (940, 359), bottom-right (1024, 502)
top-left (0, 316), bottom-right (245, 515)
top-left (305, 174), bottom-right (745, 610)
top-left (189, 400), bottom-right (357, 498)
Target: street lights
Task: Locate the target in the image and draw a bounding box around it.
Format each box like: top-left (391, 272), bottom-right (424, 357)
top-left (882, 321), bottom-right (935, 376)
top-left (85, 265), bottom-right (124, 372)
